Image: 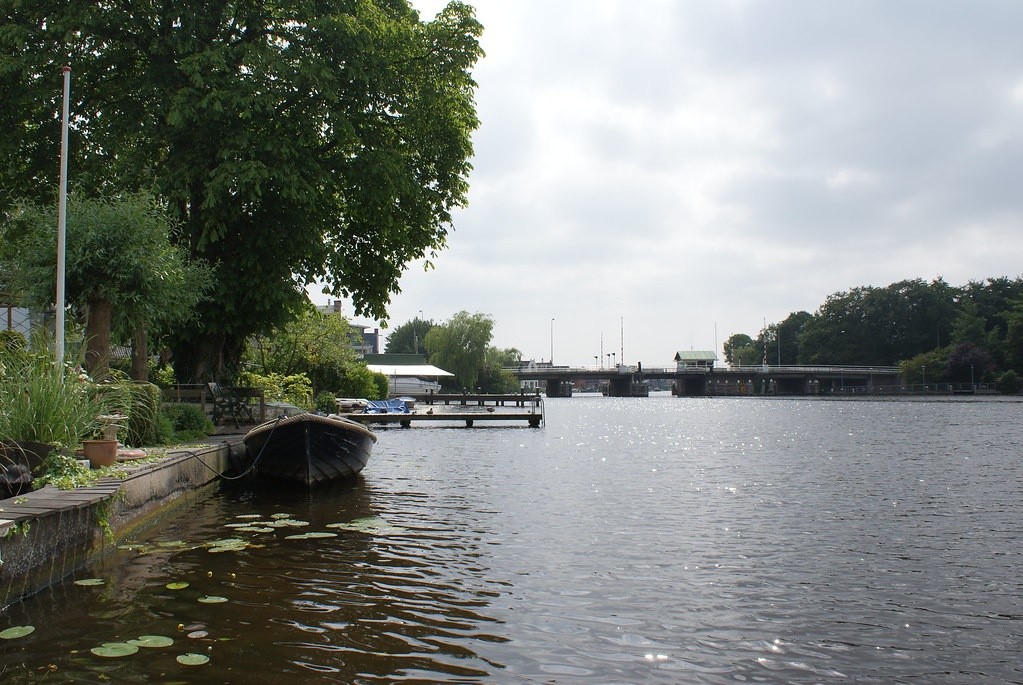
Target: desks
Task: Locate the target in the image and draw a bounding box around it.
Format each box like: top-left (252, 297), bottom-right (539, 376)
top-left (172, 387), bottom-right (265, 425)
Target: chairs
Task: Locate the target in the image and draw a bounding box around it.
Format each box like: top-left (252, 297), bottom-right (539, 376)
top-left (208, 383), bottom-right (247, 430)
top-left (228, 386), bottom-right (257, 428)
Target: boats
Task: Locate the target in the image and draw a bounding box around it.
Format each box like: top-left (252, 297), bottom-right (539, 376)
top-left (242, 411), bottom-right (378, 491)
top-left (398, 396), bottom-right (416, 407)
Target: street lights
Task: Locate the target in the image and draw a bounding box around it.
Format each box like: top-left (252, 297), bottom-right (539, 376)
top-left (612, 353), bottom-right (615, 368)
top-left (607, 354), bottom-right (610, 368)
top-left (594, 356), bottom-right (597, 369)
top-left (550, 318), bottom-right (555, 366)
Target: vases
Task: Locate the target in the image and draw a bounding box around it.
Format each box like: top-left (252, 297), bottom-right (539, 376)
top-left (81, 440), bottom-right (119, 469)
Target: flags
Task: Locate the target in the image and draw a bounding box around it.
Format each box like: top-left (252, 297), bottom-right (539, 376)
top-left (612, 353), bottom-right (615, 355)
top-left (594, 355), bottom-right (598, 358)
top-left (606, 354), bottom-right (610, 356)
